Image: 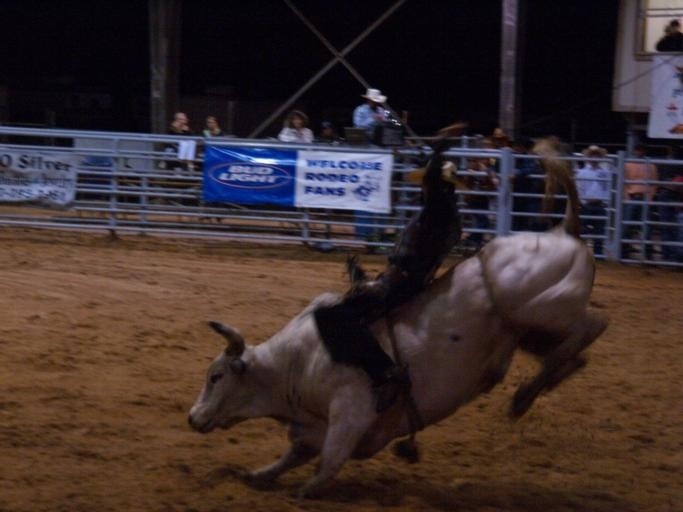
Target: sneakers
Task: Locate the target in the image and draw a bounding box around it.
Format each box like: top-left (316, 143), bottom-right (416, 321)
top-left (375, 370), bottom-right (412, 414)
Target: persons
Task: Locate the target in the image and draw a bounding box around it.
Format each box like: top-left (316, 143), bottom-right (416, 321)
top-left (656, 19), bottom-right (683, 51)
top-left (164, 89), bottom-right (408, 254)
top-left (397, 123), bottom-right (682, 265)
top-left (314, 119), bottom-right (472, 412)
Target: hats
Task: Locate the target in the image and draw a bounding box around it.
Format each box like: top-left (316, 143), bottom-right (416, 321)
top-left (582, 145), bottom-right (607, 156)
top-left (400, 160), bottom-right (471, 191)
top-left (361, 87), bottom-right (388, 103)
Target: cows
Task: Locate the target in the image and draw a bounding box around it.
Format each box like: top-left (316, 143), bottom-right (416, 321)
top-left (187, 138), bottom-right (613, 500)
